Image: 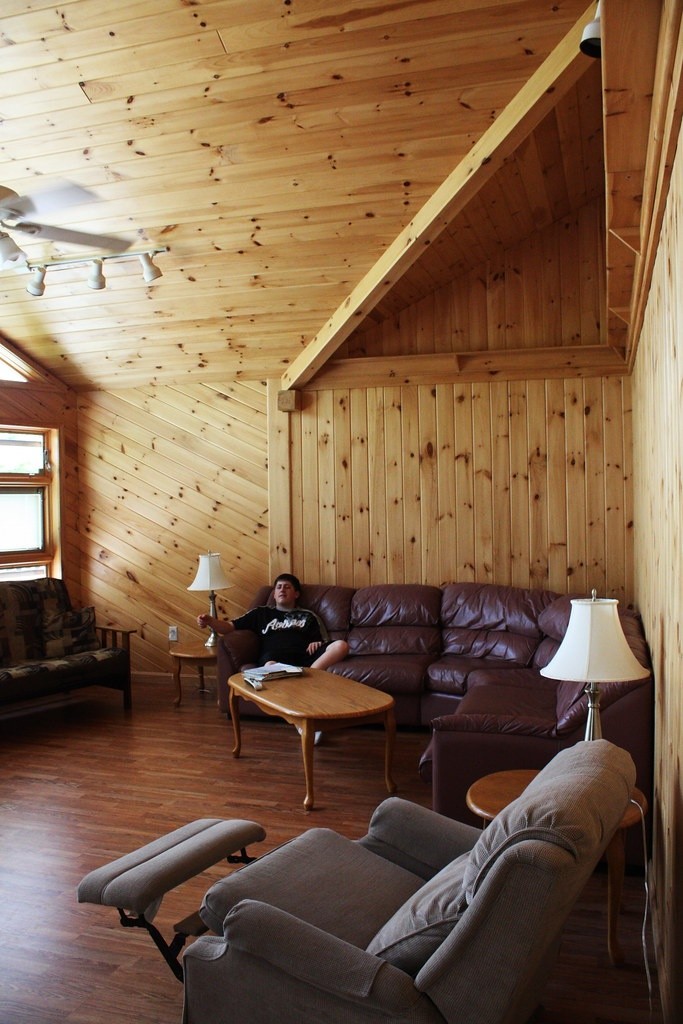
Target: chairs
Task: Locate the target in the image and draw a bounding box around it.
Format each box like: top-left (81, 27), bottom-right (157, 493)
top-left (76, 739), bottom-right (636, 1024)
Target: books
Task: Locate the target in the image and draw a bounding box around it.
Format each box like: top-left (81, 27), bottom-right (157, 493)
top-left (244, 662), bottom-right (303, 680)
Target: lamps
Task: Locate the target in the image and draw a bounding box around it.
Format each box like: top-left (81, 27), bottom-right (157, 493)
top-left (579, 0), bottom-right (602, 59)
top-left (539, 587), bottom-right (651, 741)
top-left (139, 252), bottom-right (163, 283)
top-left (26, 266), bottom-right (46, 296)
top-left (0, 230), bottom-right (26, 269)
top-left (187, 549), bottom-right (237, 646)
top-left (86, 259), bottom-right (105, 290)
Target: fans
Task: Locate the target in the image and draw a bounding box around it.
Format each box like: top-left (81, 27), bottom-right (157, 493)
top-left (0, 185), bottom-right (132, 252)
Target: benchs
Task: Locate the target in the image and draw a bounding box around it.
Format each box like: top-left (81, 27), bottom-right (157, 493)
top-left (0, 577), bottom-right (139, 711)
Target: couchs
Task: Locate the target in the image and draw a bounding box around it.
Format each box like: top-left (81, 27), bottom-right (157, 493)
top-left (215, 582), bottom-right (655, 868)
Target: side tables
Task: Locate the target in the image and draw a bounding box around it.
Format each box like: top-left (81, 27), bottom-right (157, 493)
top-left (169, 638), bottom-right (217, 708)
top-left (466, 770), bottom-right (647, 968)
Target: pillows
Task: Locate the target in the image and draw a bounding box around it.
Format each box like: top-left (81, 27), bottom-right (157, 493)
top-left (41, 606), bottom-right (101, 659)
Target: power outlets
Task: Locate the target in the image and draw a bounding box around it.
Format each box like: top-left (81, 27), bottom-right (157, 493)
top-left (168, 626), bottom-right (177, 641)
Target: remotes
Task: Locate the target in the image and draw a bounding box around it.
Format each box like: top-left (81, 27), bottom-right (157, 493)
top-left (244, 677), bottom-right (263, 691)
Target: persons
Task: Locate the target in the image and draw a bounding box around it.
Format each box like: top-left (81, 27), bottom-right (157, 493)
top-left (198, 574), bottom-right (349, 745)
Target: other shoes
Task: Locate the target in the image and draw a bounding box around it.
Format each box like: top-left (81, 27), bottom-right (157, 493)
top-left (297, 726), bottom-right (322, 745)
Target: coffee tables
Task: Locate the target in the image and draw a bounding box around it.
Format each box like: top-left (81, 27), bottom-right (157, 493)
top-left (226, 666), bottom-right (396, 811)
